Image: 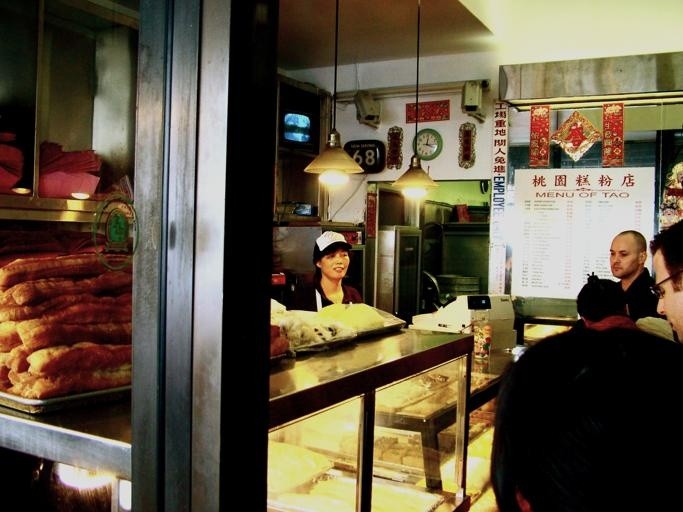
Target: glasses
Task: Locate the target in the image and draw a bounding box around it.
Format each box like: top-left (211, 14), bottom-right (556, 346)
top-left (650, 270), bottom-right (683, 299)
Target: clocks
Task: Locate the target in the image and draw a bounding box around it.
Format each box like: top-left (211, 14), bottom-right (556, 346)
top-left (412, 129), bottom-right (443, 161)
top-left (301, 2), bottom-right (364, 189)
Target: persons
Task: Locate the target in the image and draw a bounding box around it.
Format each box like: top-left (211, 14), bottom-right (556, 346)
top-left (287, 231), bottom-right (363, 311)
top-left (576, 279), bottom-right (637, 331)
top-left (609, 230), bottom-right (666, 325)
top-left (657, 201), bottom-right (679, 233)
top-left (649, 217), bottom-right (682, 346)
top-left (662, 161), bottom-right (682, 219)
top-left (491, 329), bottom-right (682, 512)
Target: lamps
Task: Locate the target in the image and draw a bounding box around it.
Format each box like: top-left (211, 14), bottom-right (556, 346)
top-left (390, 1), bottom-right (439, 199)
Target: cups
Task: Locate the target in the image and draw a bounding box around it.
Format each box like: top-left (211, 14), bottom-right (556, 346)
top-left (473, 359), bottom-right (491, 379)
top-left (473, 324), bottom-right (493, 359)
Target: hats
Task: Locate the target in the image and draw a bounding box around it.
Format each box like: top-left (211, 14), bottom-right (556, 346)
top-left (313, 231), bottom-right (352, 264)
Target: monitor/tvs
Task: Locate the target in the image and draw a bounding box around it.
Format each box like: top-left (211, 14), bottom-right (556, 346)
top-left (278, 103), bottom-right (318, 154)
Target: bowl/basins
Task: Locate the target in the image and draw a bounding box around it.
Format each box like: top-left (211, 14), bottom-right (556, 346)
top-left (277, 204), bottom-right (297, 214)
